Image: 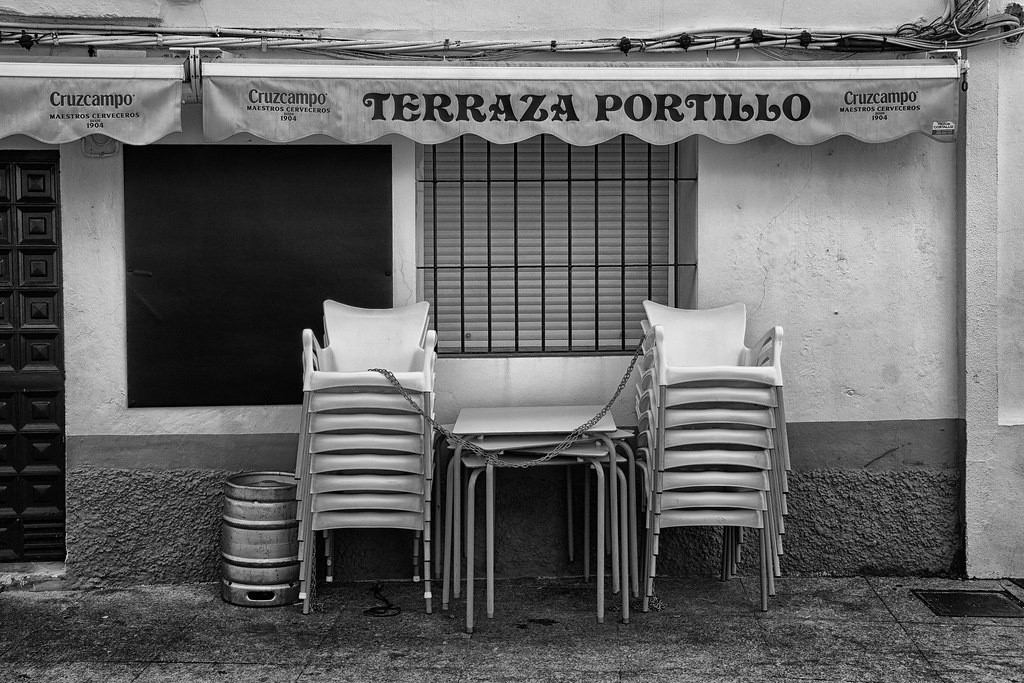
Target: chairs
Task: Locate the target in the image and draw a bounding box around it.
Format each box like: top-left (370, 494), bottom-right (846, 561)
top-left (638, 299), bottom-right (792, 617)
top-left (296, 300), bottom-right (435, 636)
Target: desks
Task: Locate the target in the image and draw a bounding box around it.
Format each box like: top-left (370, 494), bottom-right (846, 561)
top-left (438, 403), bottom-right (637, 634)
